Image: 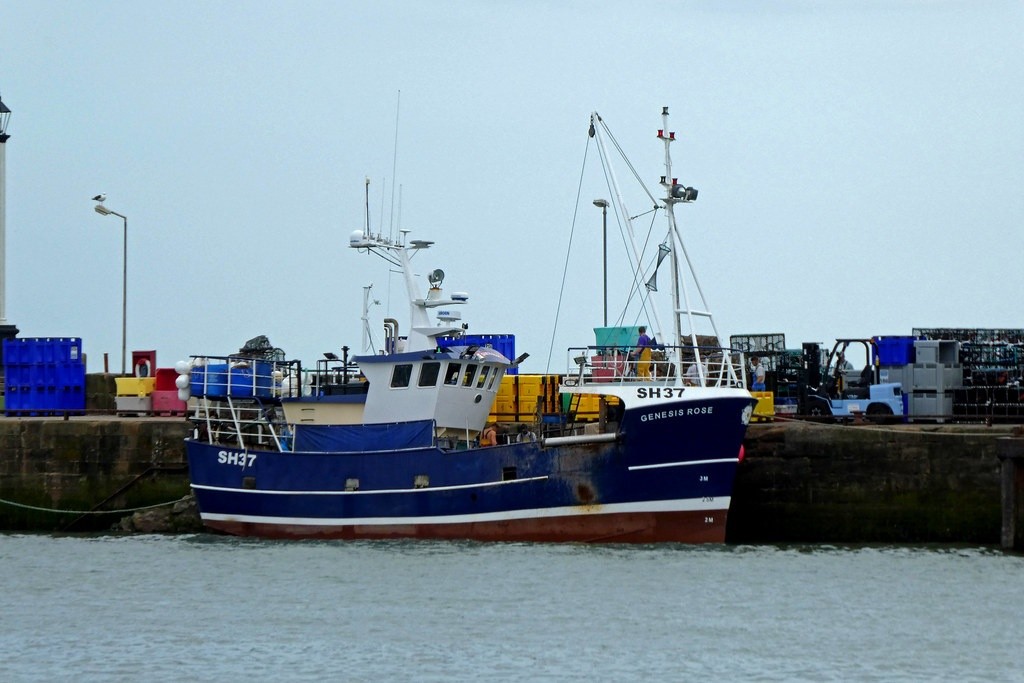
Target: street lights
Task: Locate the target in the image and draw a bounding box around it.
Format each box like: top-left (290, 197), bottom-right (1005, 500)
top-left (593, 199), bottom-right (610, 327)
top-left (0, 94), bottom-right (13, 325)
top-left (93, 204), bottom-right (128, 377)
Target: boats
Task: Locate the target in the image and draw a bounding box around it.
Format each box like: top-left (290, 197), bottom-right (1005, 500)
top-left (173, 88), bottom-right (756, 548)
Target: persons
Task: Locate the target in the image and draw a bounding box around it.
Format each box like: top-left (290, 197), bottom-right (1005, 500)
top-left (751, 356), bottom-right (765, 391)
top-left (833, 352), bottom-right (853, 391)
top-left (480, 422), bottom-right (510, 447)
top-left (516, 424), bottom-right (536, 443)
top-left (631, 326), bottom-right (652, 382)
top-left (686, 352), bottom-right (708, 387)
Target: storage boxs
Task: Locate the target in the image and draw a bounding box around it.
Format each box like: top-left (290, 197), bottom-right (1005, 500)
top-left (2, 329), bottom-right (962, 426)
